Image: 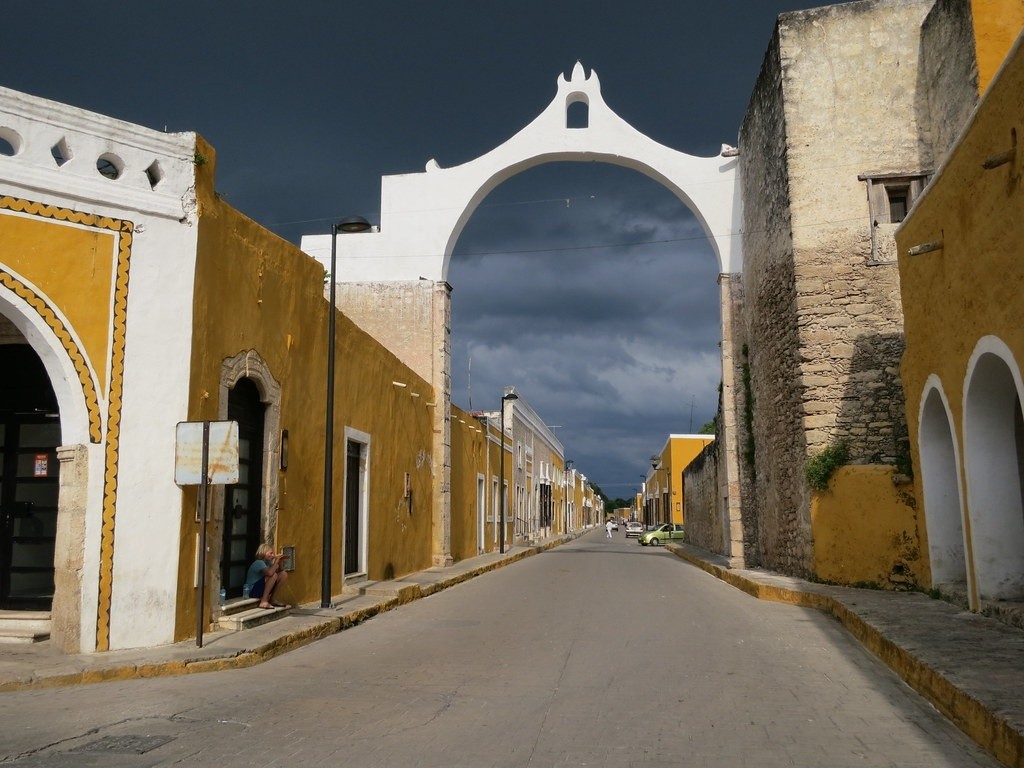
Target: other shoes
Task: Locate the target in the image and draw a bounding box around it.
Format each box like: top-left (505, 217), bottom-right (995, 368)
top-left (259, 602), bottom-right (275, 609)
top-left (270, 600), bottom-right (286, 607)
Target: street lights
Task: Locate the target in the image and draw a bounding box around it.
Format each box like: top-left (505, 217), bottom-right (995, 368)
top-left (322, 211), bottom-right (373, 606)
top-left (500, 394), bottom-right (518, 555)
top-left (565, 459), bottom-right (574, 534)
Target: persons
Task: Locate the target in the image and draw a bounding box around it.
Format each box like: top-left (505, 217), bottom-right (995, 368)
top-left (243, 543), bottom-right (287, 610)
top-left (605, 519), bottom-right (613, 539)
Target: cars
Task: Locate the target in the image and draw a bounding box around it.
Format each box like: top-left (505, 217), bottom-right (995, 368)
top-left (638, 523), bottom-right (684, 546)
top-left (606, 519), bottom-right (646, 539)
